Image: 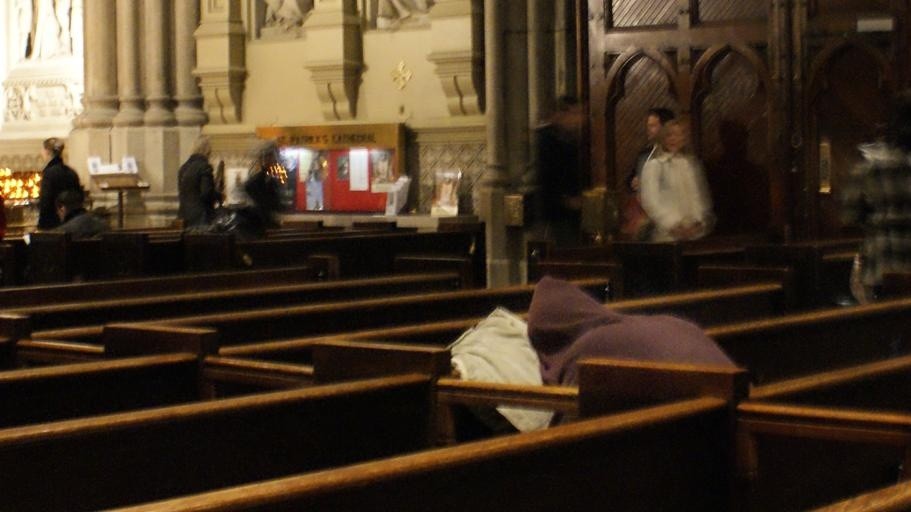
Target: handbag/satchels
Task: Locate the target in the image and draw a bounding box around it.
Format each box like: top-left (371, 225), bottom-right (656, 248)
top-left (616, 197), bottom-right (655, 242)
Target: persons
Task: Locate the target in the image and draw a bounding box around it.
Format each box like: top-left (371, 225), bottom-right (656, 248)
top-left (533, 95), bottom-right (595, 246)
top-left (628, 107), bottom-right (714, 243)
top-left (34, 136), bottom-right (287, 237)
top-left (840, 104), bottom-right (911, 305)
top-left (525, 274), bottom-right (739, 386)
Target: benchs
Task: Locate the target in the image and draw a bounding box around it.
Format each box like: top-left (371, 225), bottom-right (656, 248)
top-left (0, 216), bottom-right (910, 512)
